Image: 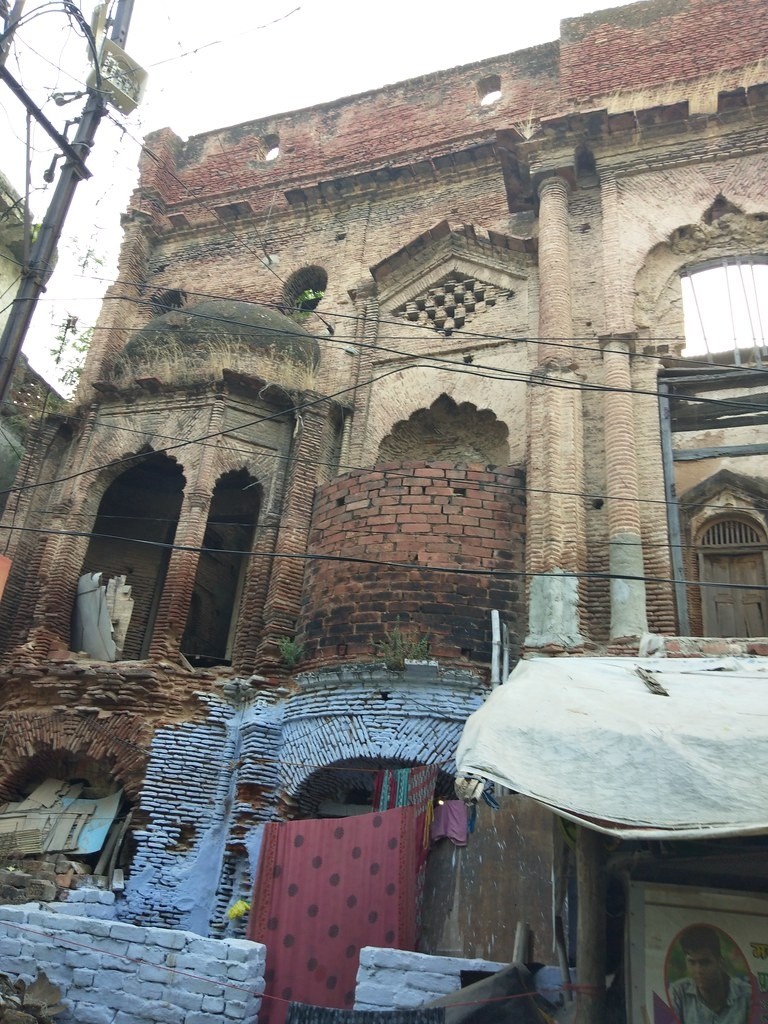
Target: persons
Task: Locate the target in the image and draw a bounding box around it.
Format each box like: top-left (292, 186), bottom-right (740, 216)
top-left (667, 925), bottom-right (754, 1024)
